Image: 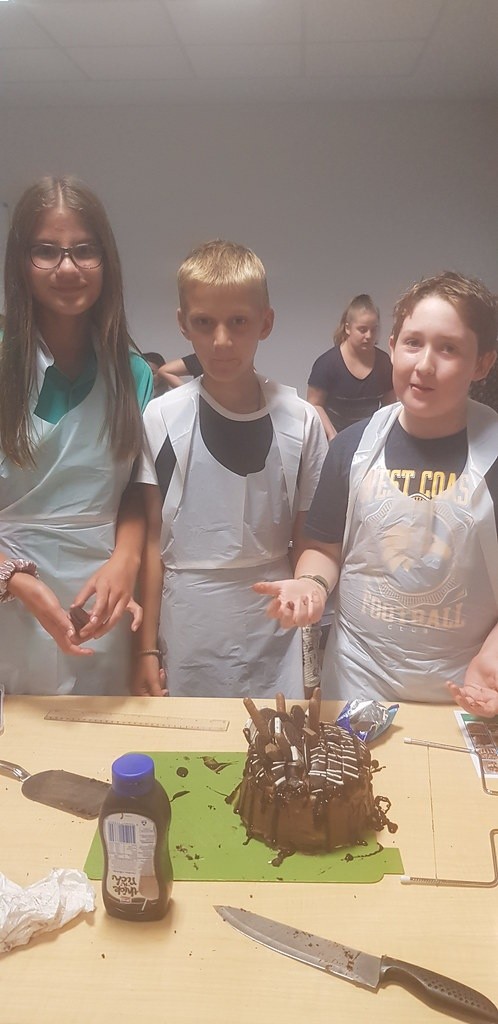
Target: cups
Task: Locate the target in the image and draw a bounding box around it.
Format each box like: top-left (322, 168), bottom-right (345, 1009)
top-left (0, 682), bottom-right (5, 733)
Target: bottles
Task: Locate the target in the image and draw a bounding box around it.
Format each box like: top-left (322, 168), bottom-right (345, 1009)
top-left (97, 753), bottom-right (174, 920)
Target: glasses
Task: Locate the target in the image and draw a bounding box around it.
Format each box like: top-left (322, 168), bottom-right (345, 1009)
top-left (29, 242), bottom-right (103, 269)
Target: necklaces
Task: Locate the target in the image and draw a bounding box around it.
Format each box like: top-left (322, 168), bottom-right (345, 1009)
top-left (201, 373), bottom-right (261, 412)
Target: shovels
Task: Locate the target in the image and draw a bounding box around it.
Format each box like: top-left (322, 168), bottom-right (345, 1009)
top-left (0, 758), bottom-right (115, 821)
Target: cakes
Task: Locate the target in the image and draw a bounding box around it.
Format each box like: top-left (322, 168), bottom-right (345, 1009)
top-left (226, 686), bottom-right (399, 868)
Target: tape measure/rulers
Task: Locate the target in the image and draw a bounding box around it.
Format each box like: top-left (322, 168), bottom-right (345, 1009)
top-left (43, 709), bottom-right (230, 732)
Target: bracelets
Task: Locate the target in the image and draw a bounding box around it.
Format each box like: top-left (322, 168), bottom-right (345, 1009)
top-left (137, 649), bottom-right (161, 655)
top-left (1, 558), bottom-right (41, 605)
top-left (297, 575), bottom-right (330, 596)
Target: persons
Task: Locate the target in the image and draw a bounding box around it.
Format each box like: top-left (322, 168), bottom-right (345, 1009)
top-left (0, 174), bottom-right (156, 696)
top-left (141, 353), bottom-right (174, 398)
top-left (306, 293), bottom-right (397, 443)
top-left (132, 239), bottom-right (330, 700)
top-left (158, 353), bottom-right (204, 388)
top-left (252, 268), bottom-right (498, 720)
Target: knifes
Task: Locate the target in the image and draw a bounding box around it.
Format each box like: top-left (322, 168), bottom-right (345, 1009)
top-left (211, 902), bottom-right (497, 1024)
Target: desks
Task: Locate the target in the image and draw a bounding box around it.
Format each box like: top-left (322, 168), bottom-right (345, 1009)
top-left (0, 692), bottom-right (497, 1024)
